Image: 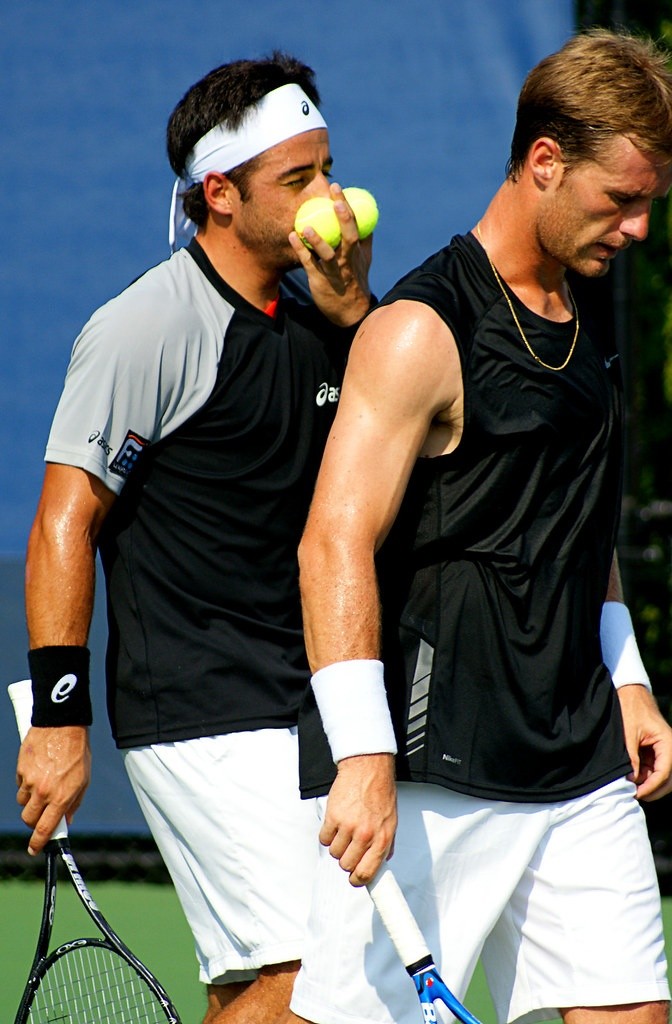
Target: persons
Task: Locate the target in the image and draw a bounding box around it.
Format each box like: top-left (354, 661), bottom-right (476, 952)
top-left (14, 26), bottom-right (672, 1024)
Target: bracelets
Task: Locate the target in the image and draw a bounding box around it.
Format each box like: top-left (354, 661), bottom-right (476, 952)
top-left (309, 660), bottom-right (398, 764)
top-left (599, 601), bottom-right (652, 693)
top-left (27, 645), bottom-right (92, 727)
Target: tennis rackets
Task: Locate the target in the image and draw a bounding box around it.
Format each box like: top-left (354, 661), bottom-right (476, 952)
top-left (316, 793), bottom-right (483, 1024)
top-left (7, 678), bottom-right (184, 1024)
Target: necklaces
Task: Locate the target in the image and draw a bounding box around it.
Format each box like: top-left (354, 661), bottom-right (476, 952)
top-left (477, 220), bottom-right (580, 371)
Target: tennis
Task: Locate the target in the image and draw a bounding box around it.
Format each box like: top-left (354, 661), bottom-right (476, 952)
top-left (294, 197), bottom-right (341, 250)
top-left (341, 186), bottom-right (380, 241)
